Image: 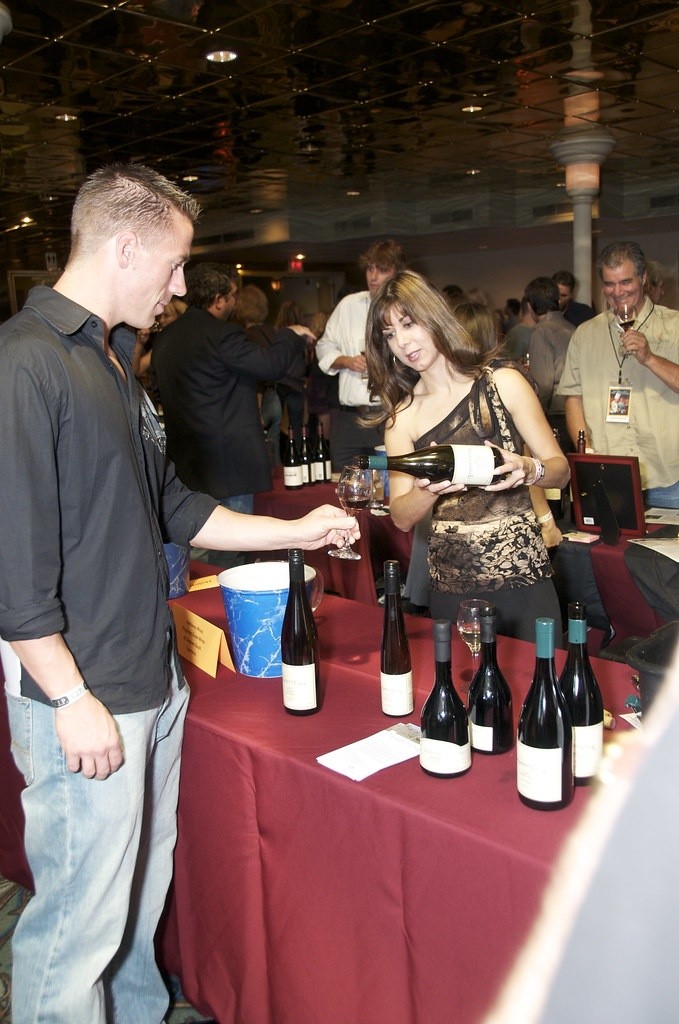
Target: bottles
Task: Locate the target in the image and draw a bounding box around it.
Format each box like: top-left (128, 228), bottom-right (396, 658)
top-left (298, 426), bottom-right (315, 486)
top-left (419, 620), bottom-right (472, 777)
top-left (466, 604), bottom-right (512, 754)
top-left (381, 560), bottom-right (414, 717)
top-left (517, 614), bottom-right (574, 812)
top-left (314, 422), bottom-right (331, 483)
top-left (358, 443), bottom-right (503, 488)
top-left (284, 426), bottom-right (303, 490)
top-left (558, 601), bottom-right (604, 786)
top-left (279, 549), bottom-right (318, 716)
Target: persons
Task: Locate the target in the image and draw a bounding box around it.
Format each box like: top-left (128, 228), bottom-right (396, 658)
top-left (644, 260), bottom-right (666, 304)
top-left (366, 269), bottom-right (571, 652)
top-left (0, 161), bottom-right (361, 1023)
top-left (610, 393), bottom-right (625, 410)
top-left (557, 240), bottom-right (679, 510)
top-left (131, 239), bottom-right (596, 612)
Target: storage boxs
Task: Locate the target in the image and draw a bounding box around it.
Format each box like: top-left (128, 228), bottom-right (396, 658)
top-left (626, 619), bottom-right (679, 719)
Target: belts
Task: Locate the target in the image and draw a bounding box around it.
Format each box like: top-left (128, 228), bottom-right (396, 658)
top-left (340, 405), bottom-right (382, 413)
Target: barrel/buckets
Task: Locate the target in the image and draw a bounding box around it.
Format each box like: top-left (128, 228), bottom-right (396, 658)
top-left (217, 563), bottom-right (317, 677)
top-left (163, 542), bottom-right (190, 599)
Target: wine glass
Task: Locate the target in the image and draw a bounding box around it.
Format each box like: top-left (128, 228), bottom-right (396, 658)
top-left (457, 602), bottom-right (488, 685)
top-left (329, 466), bottom-right (373, 559)
top-left (614, 304), bottom-right (638, 356)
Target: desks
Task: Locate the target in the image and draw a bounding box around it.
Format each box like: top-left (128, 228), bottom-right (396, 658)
top-left (0, 561), bottom-right (640, 1023)
top-left (248, 466), bottom-right (678, 660)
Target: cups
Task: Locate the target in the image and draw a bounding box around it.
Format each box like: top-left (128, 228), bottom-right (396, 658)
top-left (522, 352), bottom-right (530, 367)
top-left (358, 345), bottom-right (369, 380)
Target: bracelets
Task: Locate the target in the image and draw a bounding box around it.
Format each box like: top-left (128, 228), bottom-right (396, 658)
top-left (538, 510), bottom-right (553, 523)
top-left (51, 682), bottom-right (90, 709)
top-left (523, 457), bottom-right (542, 486)
top-left (540, 462), bottom-right (545, 479)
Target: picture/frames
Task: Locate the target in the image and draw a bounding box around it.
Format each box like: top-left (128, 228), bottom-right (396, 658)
top-left (566, 451), bottom-right (648, 539)
